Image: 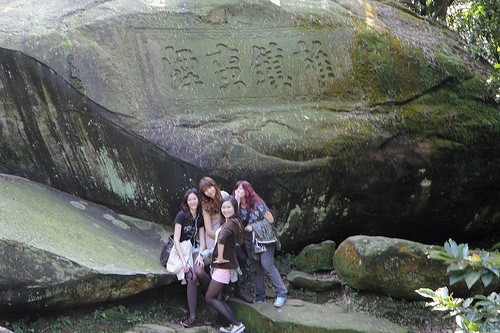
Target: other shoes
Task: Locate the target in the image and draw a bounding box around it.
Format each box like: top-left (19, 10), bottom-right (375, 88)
top-left (220, 322), bottom-right (245, 333)
top-left (254, 297), bottom-right (266, 303)
top-left (274, 296), bottom-right (287, 307)
top-left (234, 284), bottom-right (253, 303)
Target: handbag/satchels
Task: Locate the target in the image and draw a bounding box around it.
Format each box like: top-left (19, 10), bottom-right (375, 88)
top-left (160, 235), bottom-right (174, 267)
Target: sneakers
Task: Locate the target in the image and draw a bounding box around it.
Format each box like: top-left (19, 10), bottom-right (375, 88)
top-left (182, 315), bottom-right (196, 327)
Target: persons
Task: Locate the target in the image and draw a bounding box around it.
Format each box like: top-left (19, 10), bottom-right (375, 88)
top-left (198, 176), bottom-right (254, 305)
top-left (173, 188), bottom-right (229, 328)
top-left (204, 196), bottom-right (246, 333)
top-left (231, 180), bottom-right (288, 308)
